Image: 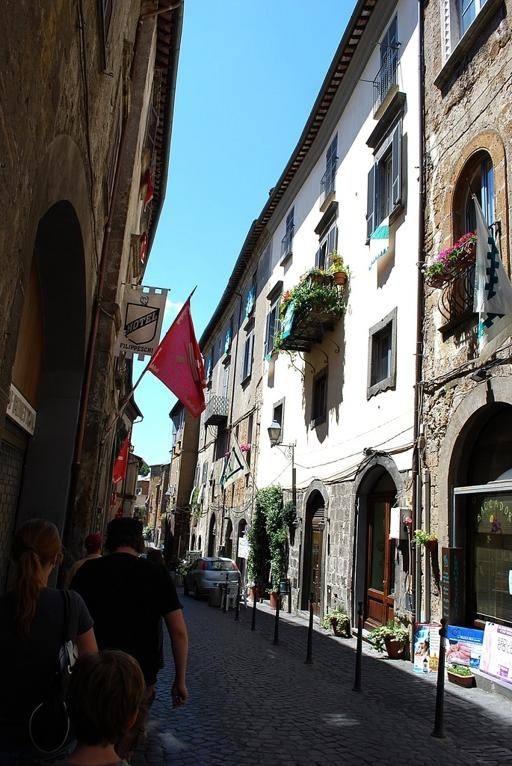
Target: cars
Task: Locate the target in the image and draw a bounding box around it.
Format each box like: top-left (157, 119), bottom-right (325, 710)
top-left (181, 555), bottom-right (246, 603)
top-left (137, 545), bottom-right (162, 562)
top-left (141, 530), bottom-right (149, 538)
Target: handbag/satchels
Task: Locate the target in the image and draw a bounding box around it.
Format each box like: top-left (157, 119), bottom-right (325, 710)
top-left (27, 586), bottom-right (79, 757)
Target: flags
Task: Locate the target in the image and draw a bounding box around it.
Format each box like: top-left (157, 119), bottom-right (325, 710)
top-left (219, 434), bottom-right (249, 489)
top-left (473, 194), bottom-right (512, 365)
top-left (146, 299), bottom-right (207, 417)
top-left (112, 433), bottom-right (128, 484)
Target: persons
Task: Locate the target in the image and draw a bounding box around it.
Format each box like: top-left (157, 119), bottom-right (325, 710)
top-left (416, 641), bottom-right (429, 656)
top-left (446, 640), bottom-right (471, 658)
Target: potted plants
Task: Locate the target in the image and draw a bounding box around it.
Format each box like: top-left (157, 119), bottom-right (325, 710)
top-left (248, 584), bottom-right (259, 601)
top-left (319, 601), bottom-right (352, 639)
top-left (446, 664), bottom-right (476, 689)
top-left (365, 619), bottom-right (410, 660)
top-left (266, 588), bottom-right (279, 608)
top-left (409, 529), bottom-right (437, 554)
top-left (290, 249), bottom-right (351, 314)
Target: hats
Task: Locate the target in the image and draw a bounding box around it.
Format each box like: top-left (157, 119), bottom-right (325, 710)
top-left (82, 531), bottom-right (103, 546)
top-left (107, 516), bottom-right (144, 541)
